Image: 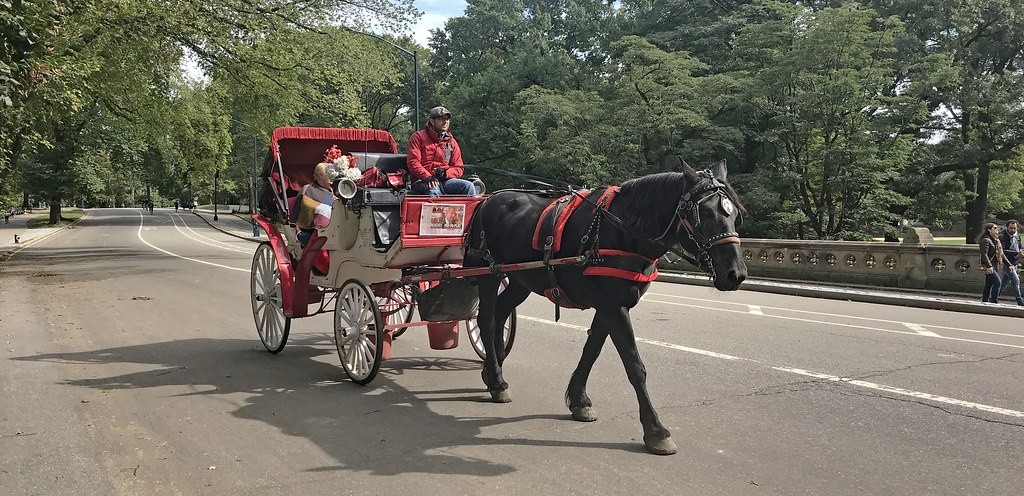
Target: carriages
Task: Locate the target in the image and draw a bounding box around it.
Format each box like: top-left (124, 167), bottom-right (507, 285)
top-left (249, 127), bottom-right (748, 456)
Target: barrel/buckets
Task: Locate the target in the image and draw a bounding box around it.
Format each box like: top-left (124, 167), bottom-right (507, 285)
top-left (360, 324), bottom-right (393, 361)
top-left (427, 321), bottom-right (460, 350)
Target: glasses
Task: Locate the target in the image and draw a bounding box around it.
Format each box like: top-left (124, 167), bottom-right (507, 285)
top-left (437, 116), bottom-right (449, 120)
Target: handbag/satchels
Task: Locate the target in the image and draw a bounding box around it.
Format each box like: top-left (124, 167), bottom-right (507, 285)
top-left (356, 167), bottom-right (387, 187)
top-left (381, 169), bottom-right (407, 197)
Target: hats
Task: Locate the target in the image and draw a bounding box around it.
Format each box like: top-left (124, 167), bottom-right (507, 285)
top-left (430, 106), bottom-right (451, 119)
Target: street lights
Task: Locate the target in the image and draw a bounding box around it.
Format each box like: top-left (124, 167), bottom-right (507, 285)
top-left (340, 22), bottom-right (418, 133)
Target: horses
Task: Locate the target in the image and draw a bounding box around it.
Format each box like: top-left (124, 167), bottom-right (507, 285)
top-left (457, 155), bottom-right (749, 455)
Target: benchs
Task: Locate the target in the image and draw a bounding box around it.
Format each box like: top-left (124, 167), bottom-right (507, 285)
top-left (365, 187), bottom-right (419, 248)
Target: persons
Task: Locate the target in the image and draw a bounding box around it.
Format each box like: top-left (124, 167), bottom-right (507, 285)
top-left (294, 163), bottom-right (340, 246)
top-left (142, 198), bottom-right (178, 215)
top-left (407, 106), bottom-right (476, 195)
top-left (999, 219), bottom-right (1024, 305)
top-left (980, 223), bottom-right (1014, 303)
